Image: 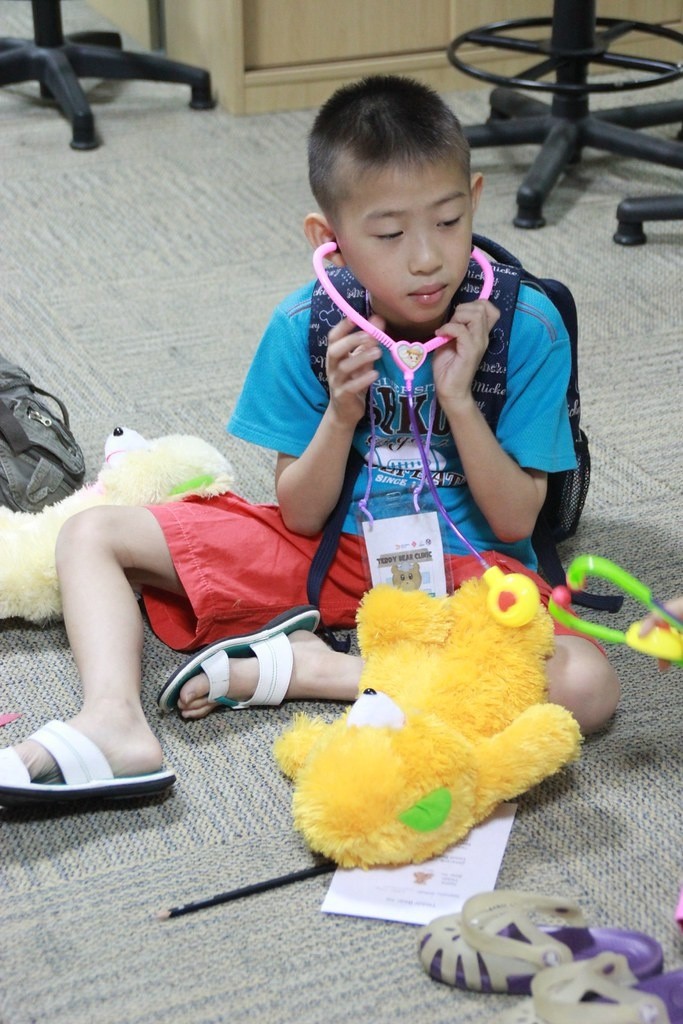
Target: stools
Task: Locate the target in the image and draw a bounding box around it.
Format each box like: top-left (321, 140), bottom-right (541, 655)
top-left (447, 0), bottom-right (683, 247)
top-left (0, 0), bottom-right (217, 152)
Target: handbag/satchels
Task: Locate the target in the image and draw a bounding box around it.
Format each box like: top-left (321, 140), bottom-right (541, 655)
top-left (0, 355), bottom-right (87, 514)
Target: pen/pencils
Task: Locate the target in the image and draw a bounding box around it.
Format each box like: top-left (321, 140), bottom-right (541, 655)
top-left (157, 861), bottom-right (338, 922)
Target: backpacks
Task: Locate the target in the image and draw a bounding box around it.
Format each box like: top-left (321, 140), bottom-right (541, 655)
top-left (308, 231), bottom-right (625, 653)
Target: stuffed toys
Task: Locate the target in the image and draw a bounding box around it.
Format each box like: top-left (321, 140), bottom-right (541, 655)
top-left (2, 420), bottom-right (233, 625)
top-left (277, 569), bottom-right (582, 867)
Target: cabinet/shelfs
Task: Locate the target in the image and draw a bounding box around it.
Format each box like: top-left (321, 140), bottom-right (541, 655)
top-left (86, 0), bottom-right (164, 52)
top-left (161, 0), bottom-right (683, 115)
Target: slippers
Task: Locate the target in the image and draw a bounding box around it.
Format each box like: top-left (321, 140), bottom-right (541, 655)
top-left (529, 949), bottom-right (683, 1024)
top-left (416, 890), bottom-right (664, 997)
top-left (155, 604), bottom-right (321, 721)
top-left (0, 720), bottom-right (176, 798)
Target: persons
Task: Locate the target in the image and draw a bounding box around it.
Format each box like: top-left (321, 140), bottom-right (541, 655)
top-left (633, 596), bottom-right (682, 669)
top-left (1, 73), bottom-right (620, 814)
